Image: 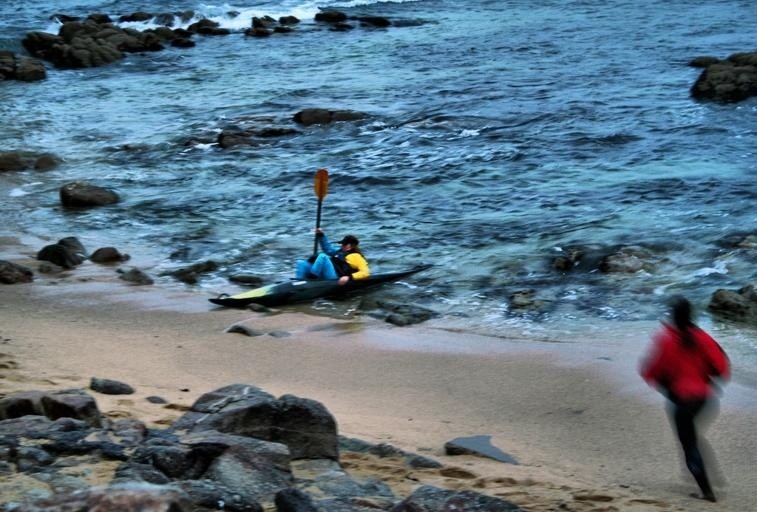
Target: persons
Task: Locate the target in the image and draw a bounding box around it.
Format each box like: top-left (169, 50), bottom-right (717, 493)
top-left (635, 295), bottom-right (733, 502)
top-left (294, 227), bottom-right (370, 286)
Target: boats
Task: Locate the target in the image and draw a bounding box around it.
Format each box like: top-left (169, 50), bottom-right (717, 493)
top-left (209, 264), bottom-right (433, 306)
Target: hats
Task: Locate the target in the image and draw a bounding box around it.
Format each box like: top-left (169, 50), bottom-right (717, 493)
top-left (337, 235), bottom-right (359, 246)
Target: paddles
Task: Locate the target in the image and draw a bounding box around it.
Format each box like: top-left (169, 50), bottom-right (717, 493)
top-left (315, 169), bottom-right (328, 257)
